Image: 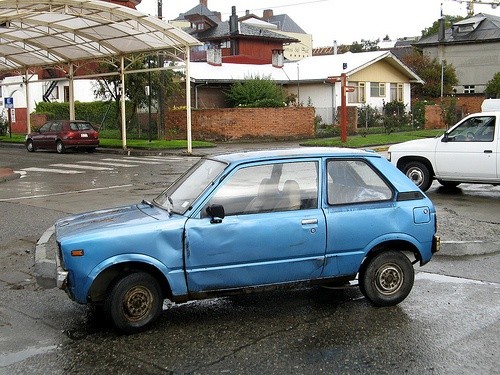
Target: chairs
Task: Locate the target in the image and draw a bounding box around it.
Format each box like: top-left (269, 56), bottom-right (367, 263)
top-left (245, 179), bottom-right (275, 210)
top-left (273, 180), bottom-right (302, 211)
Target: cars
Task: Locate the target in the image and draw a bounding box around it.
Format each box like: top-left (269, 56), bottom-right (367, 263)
top-left (25, 119), bottom-right (99, 154)
top-left (55, 146), bottom-right (441, 335)
top-left (386, 111), bottom-right (500, 192)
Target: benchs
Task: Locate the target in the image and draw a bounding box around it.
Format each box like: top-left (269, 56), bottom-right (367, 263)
top-left (312, 183), bottom-right (386, 204)
